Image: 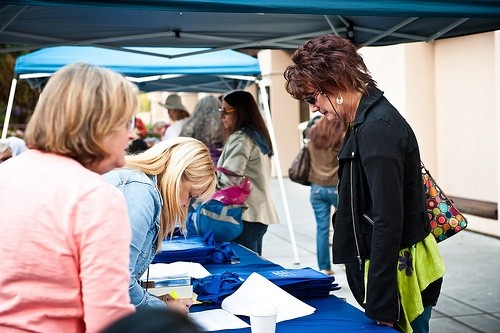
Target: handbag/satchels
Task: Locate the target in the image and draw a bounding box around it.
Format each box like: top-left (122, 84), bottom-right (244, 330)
top-left (212, 166), bottom-right (251, 204)
top-left (287, 148), bottom-right (311, 187)
top-left (420, 159), bottom-right (467, 243)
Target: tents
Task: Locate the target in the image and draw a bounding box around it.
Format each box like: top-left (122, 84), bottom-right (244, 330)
top-left (1, 46), bottom-right (300, 265)
top-left (0, 0), bottom-right (500, 60)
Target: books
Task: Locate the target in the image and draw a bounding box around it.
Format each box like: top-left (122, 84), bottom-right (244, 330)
top-left (138, 261), bottom-right (317, 331)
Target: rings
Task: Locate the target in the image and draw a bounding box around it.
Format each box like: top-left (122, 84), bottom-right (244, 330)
top-left (377, 322), bottom-right (382, 326)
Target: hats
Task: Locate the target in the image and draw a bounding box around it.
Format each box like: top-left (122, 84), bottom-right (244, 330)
top-left (157, 94), bottom-right (191, 117)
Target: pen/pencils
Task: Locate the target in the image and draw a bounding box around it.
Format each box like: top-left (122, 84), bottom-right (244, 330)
top-left (168, 290), bottom-right (181, 301)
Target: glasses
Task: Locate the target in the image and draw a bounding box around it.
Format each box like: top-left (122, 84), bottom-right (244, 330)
top-left (126, 113), bottom-right (137, 133)
top-left (218, 108), bottom-right (237, 114)
top-left (304, 89), bottom-right (319, 104)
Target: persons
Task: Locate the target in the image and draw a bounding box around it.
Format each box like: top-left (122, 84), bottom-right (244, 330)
top-left (215, 89), bottom-right (279, 258)
top-left (283, 34), bottom-right (444, 333)
top-left (124, 93), bottom-right (231, 167)
top-left (100, 135), bottom-right (217, 317)
top-left (303, 114), bottom-right (349, 276)
top-left (0, 62), bottom-right (139, 333)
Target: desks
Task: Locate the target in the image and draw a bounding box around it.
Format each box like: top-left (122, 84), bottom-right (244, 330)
top-left (139, 232), bottom-right (401, 333)
